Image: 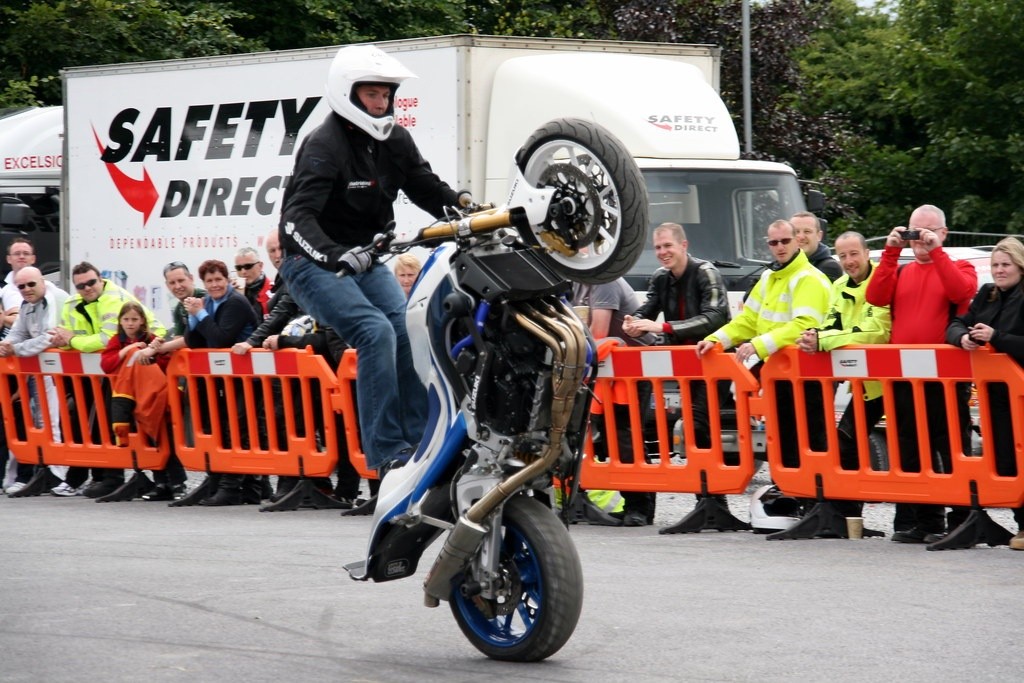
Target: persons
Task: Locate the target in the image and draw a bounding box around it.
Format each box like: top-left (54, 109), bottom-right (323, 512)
top-left (0, 229), bottom-right (381, 508)
top-left (585, 272), bottom-right (659, 526)
top-left (865, 204), bottom-right (977, 546)
top-left (622, 221), bottom-right (736, 513)
top-left (278, 44), bottom-right (474, 476)
top-left (796, 231), bottom-right (893, 541)
top-left (947, 236), bottom-right (1024, 550)
top-left (394, 253), bottom-right (422, 298)
top-left (695, 211), bottom-right (840, 535)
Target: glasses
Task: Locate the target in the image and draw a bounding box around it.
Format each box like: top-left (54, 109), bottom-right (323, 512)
top-left (234, 262), bottom-right (260, 273)
top-left (765, 237), bottom-right (794, 248)
top-left (164, 260), bottom-right (186, 280)
top-left (16, 280), bottom-right (40, 291)
top-left (9, 251), bottom-right (33, 258)
top-left (75, 278), bottom-right (100, 290)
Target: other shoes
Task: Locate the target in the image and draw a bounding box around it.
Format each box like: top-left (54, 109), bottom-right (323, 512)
top-left (378, 443), bottom-right (419, 479)
top-left (312, 477), bottom-right (336, 498)
top-left (556, 486), bottom-right (1023, 550)
top-left (330, 474), bottom-right (362, 504)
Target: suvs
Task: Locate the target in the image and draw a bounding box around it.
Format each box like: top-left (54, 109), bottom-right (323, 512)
top-left (829, 244), bottom-right (1001, 513)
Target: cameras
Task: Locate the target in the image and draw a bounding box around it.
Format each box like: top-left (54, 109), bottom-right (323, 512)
top-left (968, 327), bottom-right (985, 346)
top-left (898, 230), bottom-right (920, 241)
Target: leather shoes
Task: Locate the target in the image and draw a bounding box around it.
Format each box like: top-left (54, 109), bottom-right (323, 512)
top-left (0, 463), bottom-right (301, 506)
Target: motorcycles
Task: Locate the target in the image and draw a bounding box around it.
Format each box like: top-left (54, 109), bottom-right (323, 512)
top-left (327, 115), bottom-right (650, 662)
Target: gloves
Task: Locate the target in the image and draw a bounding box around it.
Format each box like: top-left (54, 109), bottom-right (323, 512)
top-left (336, 245), bottom-right (372, 276)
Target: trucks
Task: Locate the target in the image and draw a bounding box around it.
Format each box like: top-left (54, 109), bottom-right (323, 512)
top-left (59, 31), bottom-right (816, 349)
top-left (0, 103), bottom-right (65, 298)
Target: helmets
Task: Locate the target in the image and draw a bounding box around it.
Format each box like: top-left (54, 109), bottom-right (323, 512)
top-left (748, 485), bottom-right (800, 530)
top-left (325, 44), bottom-right (418, 141)
